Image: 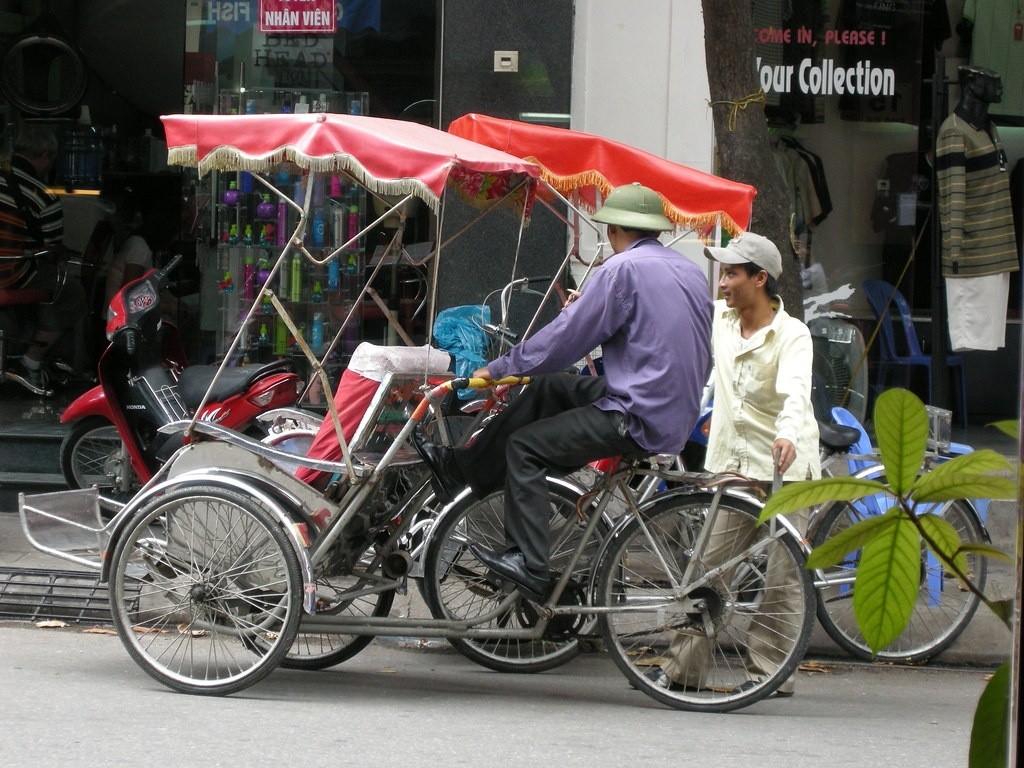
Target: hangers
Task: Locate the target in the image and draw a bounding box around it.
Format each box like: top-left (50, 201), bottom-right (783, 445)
top-left (767, 124), bottom-right (797, 152)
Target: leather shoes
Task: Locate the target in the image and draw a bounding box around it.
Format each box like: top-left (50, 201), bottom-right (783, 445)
top-left (469, 543), bottom-right (551, 603)
top-left (412, 423), bottom-right (465, 505)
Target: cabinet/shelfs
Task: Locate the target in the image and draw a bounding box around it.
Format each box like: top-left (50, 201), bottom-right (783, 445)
top-left (218, 88), bottom-right (369, 365)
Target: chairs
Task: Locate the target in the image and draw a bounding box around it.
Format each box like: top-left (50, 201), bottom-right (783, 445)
top-left (863, 279), bottom-right (968, 436)
top-left (830, 407), bottom-right (987, 604)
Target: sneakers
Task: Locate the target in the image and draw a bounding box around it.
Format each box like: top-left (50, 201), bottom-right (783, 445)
top-left (5, 360), bottom-right (54, 397)
top-left (733, 678), bottom-right (794, 701)
top-left (628, 671), bottom-right (700, 690)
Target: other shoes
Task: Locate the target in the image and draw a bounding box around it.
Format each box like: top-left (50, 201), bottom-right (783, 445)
top-left (82, 370), bottom-right (96, 382)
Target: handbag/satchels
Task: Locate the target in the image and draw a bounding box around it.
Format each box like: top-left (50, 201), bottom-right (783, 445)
top-left (433, 304), bottom-right (492, 401)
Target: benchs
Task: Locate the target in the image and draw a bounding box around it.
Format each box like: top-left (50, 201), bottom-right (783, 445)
top-left (161, 341), bottom-right (452, 593)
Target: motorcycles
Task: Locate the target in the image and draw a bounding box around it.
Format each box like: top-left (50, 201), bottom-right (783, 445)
top-left (58, 253), bottom-right (333, 536)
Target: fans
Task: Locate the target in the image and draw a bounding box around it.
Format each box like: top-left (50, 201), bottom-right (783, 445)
top-left (811, 335), bottom-right (851, 417)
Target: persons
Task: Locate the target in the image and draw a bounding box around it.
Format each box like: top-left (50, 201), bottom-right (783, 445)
top-left (936, 66), bottom-right (1019, 352)
top-left (0, 124), bottom-right (81, 396)
top-left (100, 206), bottom-right (182, 340)
top-left (401, 181), bottom-right (715, 603)
top-left (628, 231), bottom-right (821, 697)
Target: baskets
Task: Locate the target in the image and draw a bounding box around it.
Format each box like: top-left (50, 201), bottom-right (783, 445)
top-left (440, 388), bottom-right (481, 416)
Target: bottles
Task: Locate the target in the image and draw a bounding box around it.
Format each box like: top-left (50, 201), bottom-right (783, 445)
top-left (222, 170), bottom-right (358, 406)
top-left (293, 95), bottom-right (309, 113)
top-left (351, 94), bottom-right (361, 115)
top-left (313, 93), bottom-right (330, 113)
top-left (244, 93), bottom-right (256, 114)
top-left (282, 101), bottom-right (292, 114)
top-left (229, 101), bottom-right (238, 114)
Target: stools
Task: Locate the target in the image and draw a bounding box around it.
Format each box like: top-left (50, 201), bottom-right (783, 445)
top-left (0, 286), bottom-right (47, 397)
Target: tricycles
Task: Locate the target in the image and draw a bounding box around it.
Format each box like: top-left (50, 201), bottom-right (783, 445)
top-left (16, 112), bottom-right (988, 720)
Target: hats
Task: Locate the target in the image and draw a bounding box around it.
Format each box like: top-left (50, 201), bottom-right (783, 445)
top-left (591, 182), bottom-right (673, 232)
top-left (704, 231), bottom-right (783, 281)
